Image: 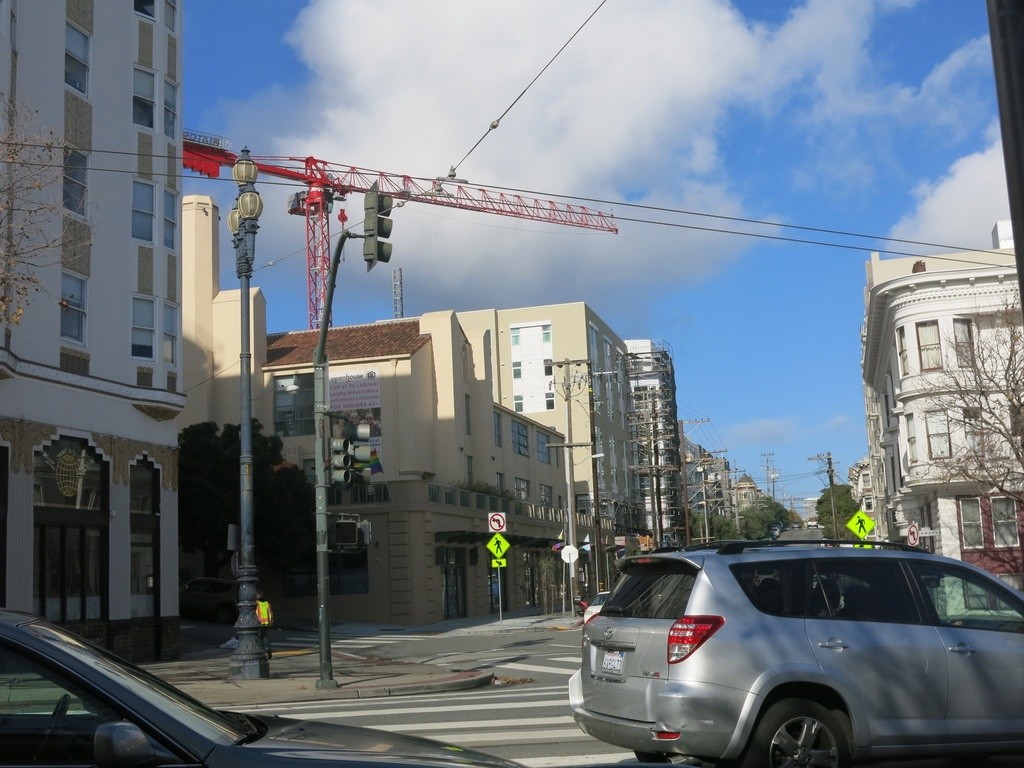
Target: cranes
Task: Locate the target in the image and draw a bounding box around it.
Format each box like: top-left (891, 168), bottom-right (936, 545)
top-left (183, 127), bottom-right (620, 237)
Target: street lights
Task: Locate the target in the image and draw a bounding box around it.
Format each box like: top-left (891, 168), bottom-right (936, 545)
top-left (225, 144), bottom-right (271, 679)
top-left (566, 453), bottom-right (607, 617)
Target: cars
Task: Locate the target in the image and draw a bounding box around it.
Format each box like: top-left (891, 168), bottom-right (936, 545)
top-left (178, 577), bottom-right (240, 625)
top-left (0, 607), bottom-right (528, 768)
top-left (584, 591), bottom-right (610, 625)
top-left (791, 521), bottom-right (803, 528)
top-left (768, 521), bottom-right (784, 538)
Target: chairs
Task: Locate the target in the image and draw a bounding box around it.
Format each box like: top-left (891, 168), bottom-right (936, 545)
top-left (757, 578), bottom-right (779, 602)
top-left (815, 573), bottom-right (840, 608)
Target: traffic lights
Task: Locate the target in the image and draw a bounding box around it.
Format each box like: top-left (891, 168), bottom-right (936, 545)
top-left (329, 415), bottom-right (370, 485)
top-left (363, 179), bottom-right (394, 273)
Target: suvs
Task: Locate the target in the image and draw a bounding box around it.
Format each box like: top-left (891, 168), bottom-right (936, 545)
top-left (566, 538), bottom-right (1023, 768)
top-left (807, 516), bottom-right (819, 528)
